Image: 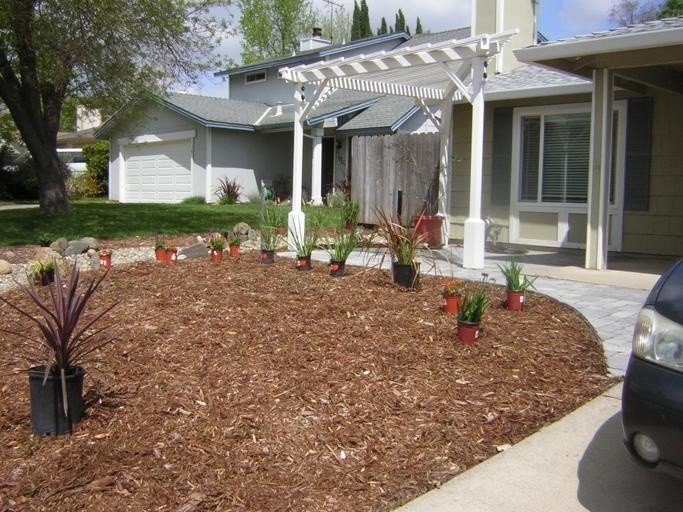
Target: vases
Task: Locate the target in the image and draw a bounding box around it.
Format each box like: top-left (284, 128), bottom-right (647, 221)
top-left (415, 215), bottom-right (446, 248)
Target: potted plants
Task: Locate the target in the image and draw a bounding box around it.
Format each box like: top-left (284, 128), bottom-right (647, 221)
top-left (259, 208), bottom-right (288, 264)
top-left (344, 201), bottom-right (360, 229)
top-left (318, 227), bottom-right (357, 277)
top-left (31, 256), bottom-right (66, 287)
top-left (154, 237), bottom-right (177, 264)
top-left (282, 216), bottom-right (321, 270)
top-left (98, 247), bottom-right (113, 267)
top-left (210, 234), bottom-right (241, 263)
top-left (360, 206), bottom-right (453, 289)
top-left (1, 256), bottom-right (123, 438)
top-left (442, 286), bottom-right (462, 314)
top-left (498, 259), bottom-right (539, 311)
top-left (456, 288), bottom-right (491, 345)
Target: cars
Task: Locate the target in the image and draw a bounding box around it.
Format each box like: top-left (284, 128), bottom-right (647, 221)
top-left (623, 258), bottom-right (681, 480)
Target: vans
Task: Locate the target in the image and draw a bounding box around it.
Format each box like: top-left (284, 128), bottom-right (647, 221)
top-left (54, 147), bottom-right (88, 180)
